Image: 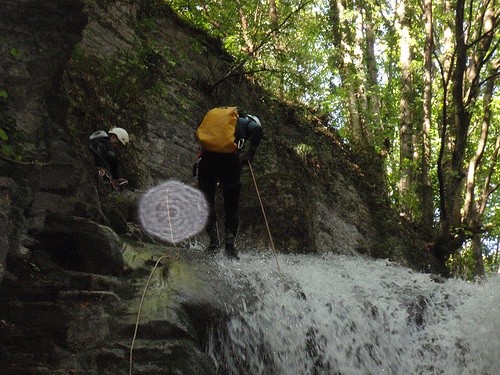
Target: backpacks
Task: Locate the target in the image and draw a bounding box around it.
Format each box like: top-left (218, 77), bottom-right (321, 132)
top-left (196, 106), bottom-right (245, 154)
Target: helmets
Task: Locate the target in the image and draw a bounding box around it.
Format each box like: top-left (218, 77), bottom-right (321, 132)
top-left (108, 128), bottom-right (129, 146)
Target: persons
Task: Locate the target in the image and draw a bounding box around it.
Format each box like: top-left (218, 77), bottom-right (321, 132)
top-left (87, 127), bottom-right (141, 242)
top-left (197, 113), bottom-right (264, 261)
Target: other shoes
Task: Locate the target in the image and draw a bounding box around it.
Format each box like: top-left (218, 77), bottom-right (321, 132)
top-left (205, 243), bottom-right (219, 257)
top-left (225, 240), bottom-right (241, 261)
